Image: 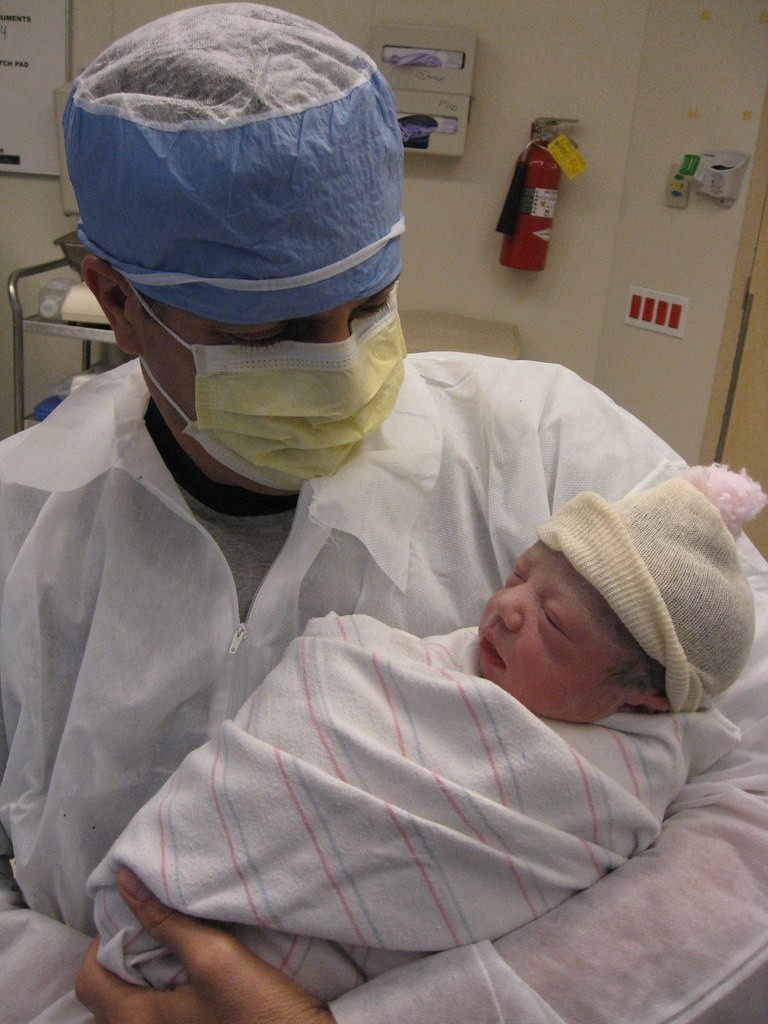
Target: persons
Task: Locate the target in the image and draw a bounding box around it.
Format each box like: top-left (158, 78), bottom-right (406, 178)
top-left (0, 3), bottom-right (768, 1024)
top-left (85, 463), bottom-right (768, 1002)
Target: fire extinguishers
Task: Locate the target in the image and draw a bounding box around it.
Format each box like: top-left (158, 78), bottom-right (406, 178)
top-left (494, 118), bottom-right (581, 272)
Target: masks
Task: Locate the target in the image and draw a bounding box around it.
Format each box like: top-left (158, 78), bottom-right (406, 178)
top-left (127, 281), bottom-right (406, 494)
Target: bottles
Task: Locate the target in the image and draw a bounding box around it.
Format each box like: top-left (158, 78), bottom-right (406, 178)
top-left (37, 278), bottom-right (79, 320)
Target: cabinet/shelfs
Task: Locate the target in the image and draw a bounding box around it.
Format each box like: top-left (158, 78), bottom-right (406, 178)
top-left (7, 258), bottom-right (143, 434)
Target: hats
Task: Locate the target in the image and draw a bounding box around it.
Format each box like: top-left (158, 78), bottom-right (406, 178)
top-left (60, 3), bottom-right (406, 326)
top-left (537, 462), bottom-right (768, 713)
